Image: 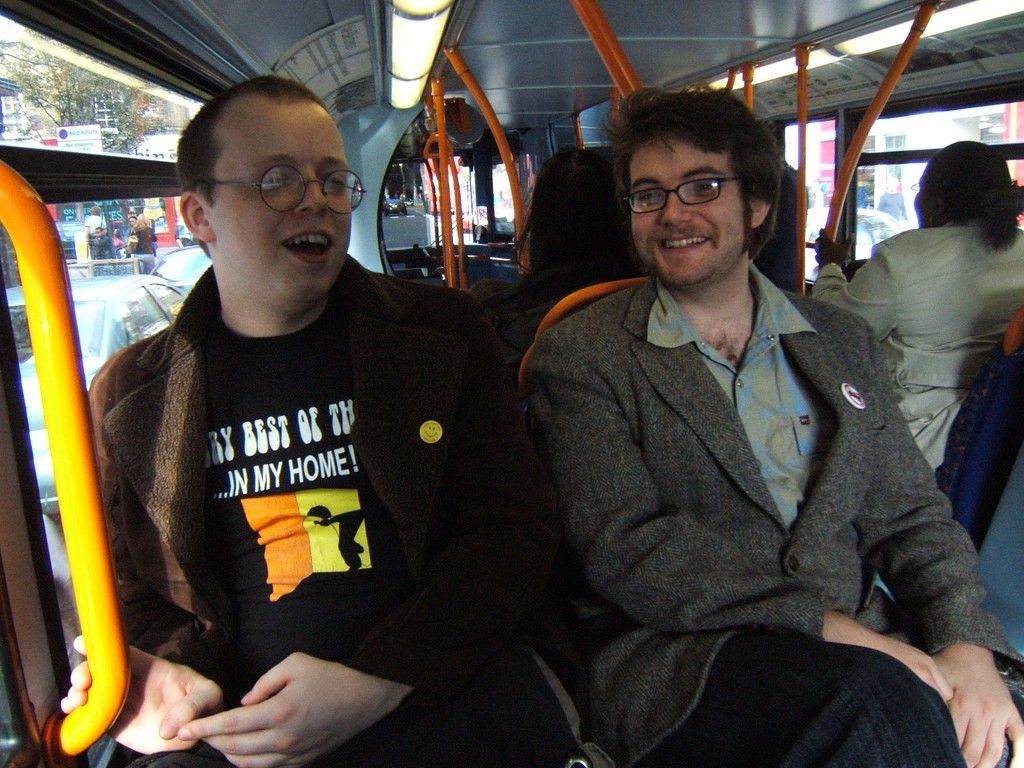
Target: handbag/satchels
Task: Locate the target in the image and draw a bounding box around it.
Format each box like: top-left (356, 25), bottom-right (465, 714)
top-left (127, 228), bottom-right (139, 253)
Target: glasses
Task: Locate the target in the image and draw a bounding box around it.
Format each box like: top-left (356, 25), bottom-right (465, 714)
top-left (622, 176), bottom-right (743, 214)
top-left (183, 163), bottom-right (366, 214)
top-left (911, 183), bottom-right (921, 191)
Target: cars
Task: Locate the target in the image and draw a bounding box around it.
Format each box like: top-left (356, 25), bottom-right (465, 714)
top-left (54, 221), bottom-right (90, 259)
top-left (384, 199), bottom-right (408, 217)
top-left (401, 198), bottom-right (415, 206)
top-left (4, 275), bottom-right (191, 517)
top-left (148, 244), bottom-right (214, 299)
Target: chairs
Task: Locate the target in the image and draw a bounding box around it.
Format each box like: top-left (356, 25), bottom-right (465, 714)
top-left (388, 245), bottom-right (1024, 526)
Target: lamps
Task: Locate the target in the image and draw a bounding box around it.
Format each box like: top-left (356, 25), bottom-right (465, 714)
top-left (381, 0), bottom-right (454, 111)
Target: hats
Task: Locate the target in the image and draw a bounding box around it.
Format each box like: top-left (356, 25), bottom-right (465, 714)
top-left (113, 229), bottom-right (120, 236)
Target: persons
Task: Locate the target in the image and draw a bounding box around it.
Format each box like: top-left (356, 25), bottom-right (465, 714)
top-left (521, 86), bottom-right (1024, 768)
top-left (85, 205), bottom-right (158, 274)
top-left (876, 175), bottom-right (908, 221)
top-left (174, 226), bottom-right (193, 248)
top-left (480, 147), bottom-right (650, 391)
top-left (753, 163), bottom-right (798, 293)
top-left (812, 141), bottom-right (1024, 472)
top-left (60, 74), bottom-right (564, 768)
